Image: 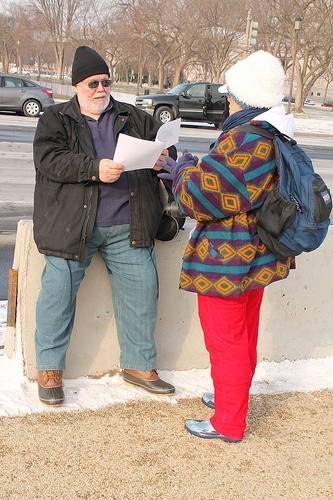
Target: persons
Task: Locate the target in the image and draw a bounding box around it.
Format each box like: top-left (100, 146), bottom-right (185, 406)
top-left (157, 51), bottom-right (296, 445)
top-left (34, 45), bottom-right (177, 412)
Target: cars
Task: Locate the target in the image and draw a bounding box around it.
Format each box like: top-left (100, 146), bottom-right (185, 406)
top-left (8, 68), bottom-right (72, 80)
top-left (321, 101), bottom-right (333, 107)
top-left (0, 74), bottom-right (56, 119)
top-left (283, 95), bottom-right (315, 105)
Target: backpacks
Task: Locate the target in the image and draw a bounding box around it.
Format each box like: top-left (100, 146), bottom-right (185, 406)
top-left (238, 127), bottom-right (333, 260)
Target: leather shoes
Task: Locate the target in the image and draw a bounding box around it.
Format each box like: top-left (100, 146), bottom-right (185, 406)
top-left (184, 420), bottom-right (240, 444)
top-left (202, 392), bottom-right (216, 408)
top-left (37, 370), bottom-right (64, 406)
top-left (124, 369), bottom-right (175, 396)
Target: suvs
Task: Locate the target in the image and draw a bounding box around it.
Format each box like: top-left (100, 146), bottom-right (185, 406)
top-left (134, 81), bottom-right (230, 129)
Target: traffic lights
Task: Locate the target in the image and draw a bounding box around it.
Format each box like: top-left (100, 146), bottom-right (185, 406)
top-left (249, 20), bottom-right (258, 47)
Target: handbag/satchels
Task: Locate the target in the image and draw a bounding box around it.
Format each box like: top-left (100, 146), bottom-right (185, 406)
top-left (154, 178), bottom-right (186, 241)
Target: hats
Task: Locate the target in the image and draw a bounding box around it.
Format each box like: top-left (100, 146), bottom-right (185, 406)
top-left (71, 45), bottom-right (110, 85)
top-left (216, 51), bottom-right (286, 111)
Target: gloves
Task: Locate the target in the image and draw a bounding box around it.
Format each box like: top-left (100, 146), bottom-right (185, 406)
top-left (157, 155), bottom-right (196, 181)
top-left (179, 150), bottom-right (199, 164)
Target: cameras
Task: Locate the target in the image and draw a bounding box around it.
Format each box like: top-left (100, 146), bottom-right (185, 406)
top-left (166, 202), bottom-right (189, 218)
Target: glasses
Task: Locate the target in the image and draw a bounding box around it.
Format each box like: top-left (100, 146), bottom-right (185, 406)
top-left (85, 80), bottom-right (111, 88)
